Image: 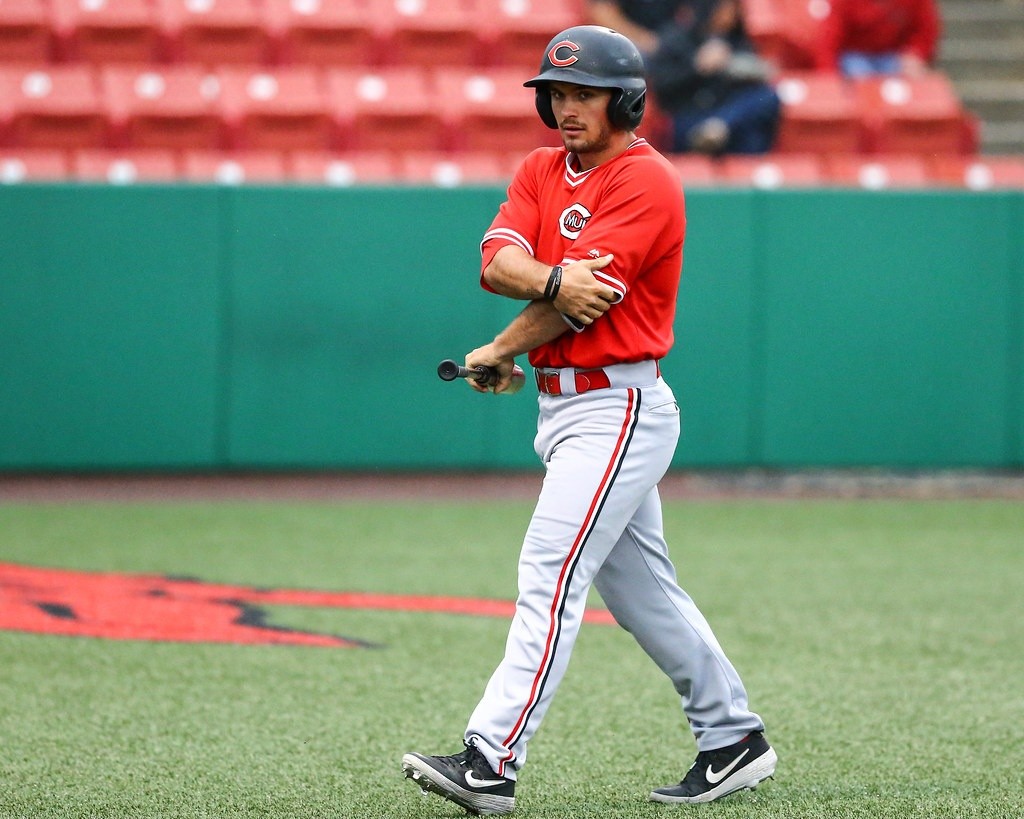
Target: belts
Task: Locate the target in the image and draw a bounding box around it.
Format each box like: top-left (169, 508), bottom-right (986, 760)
top-left (532, 359), bottom-right (662, 393)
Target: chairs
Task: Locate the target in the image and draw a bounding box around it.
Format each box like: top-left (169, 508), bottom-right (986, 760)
top-left (0, 0), bottom-right (1024, 190)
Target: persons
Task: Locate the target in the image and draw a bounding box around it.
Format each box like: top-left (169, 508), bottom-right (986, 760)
top-left (401, 23), bottom-right (745, 816)
top-left (576, 1), bottom-right (940, 157)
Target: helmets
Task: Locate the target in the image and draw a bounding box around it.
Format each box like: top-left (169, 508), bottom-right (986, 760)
top-left (521, 24), bottom-right (647, 132)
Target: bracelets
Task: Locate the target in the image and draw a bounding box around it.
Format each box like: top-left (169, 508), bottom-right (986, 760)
top-left (544, 266), bottom-right (562, 303)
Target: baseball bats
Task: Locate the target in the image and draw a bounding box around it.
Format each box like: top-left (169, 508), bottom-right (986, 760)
top-left (437, 359), bottom-right (527, 395)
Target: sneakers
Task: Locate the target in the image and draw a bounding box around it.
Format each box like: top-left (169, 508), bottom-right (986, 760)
top-left (649, 731), bottom-right (779, 806)
top-left (402, 743), bottom-right (517, 815)
